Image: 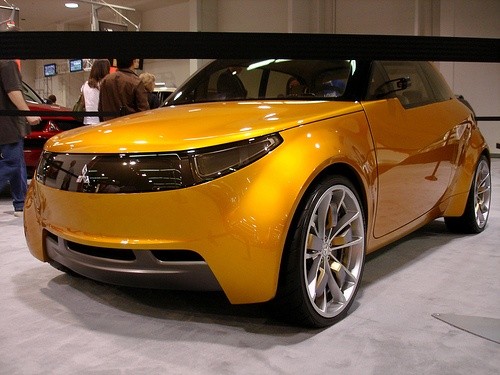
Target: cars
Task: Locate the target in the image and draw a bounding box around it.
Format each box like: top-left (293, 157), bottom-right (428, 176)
top-left (22, 57), bottom-right (491, 328)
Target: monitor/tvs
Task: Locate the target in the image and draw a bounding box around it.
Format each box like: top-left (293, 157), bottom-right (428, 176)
top-left (70, 59), bottom-right (82, 72)
top-left (44, 64), bottom-right (56, 77)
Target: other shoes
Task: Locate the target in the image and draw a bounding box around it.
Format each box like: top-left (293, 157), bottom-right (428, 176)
top-left (13, 207), bottom-right (23, 217)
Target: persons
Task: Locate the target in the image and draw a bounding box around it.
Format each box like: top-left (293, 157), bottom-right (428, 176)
top-left (49, 95), bottom-right (56, 103)
top-left (278, 79), bottom-right (348, 99)
top-left (0, 60), bottom-right (42, 218)
top-left (97, 59), bottom-right (150, 122)
top-left (80, 59), bottom-right (112, 126)
top-left (218, 68), bottom-right (248, 99)
top-left (138, 73), bottom-right (161, 112)
